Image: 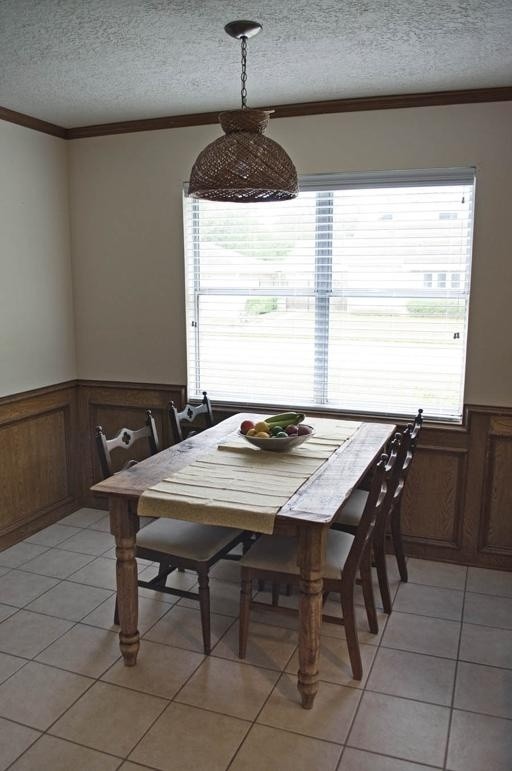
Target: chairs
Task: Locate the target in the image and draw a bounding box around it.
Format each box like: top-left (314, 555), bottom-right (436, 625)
top-left (94, 410), bottom-right (252, 658)
top-left (168, 390), bottom-right (214, 439)
top-left (288, 408), bottom-right (424, 617)
top-left (236, 452), bottom-right (381, 680)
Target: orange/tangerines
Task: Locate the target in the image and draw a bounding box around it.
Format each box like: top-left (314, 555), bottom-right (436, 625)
top-left (271, 425), bottom-right (288, 438)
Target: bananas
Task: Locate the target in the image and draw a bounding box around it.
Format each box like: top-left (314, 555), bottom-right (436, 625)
top-left (265, 411), bottom-right (305, 428)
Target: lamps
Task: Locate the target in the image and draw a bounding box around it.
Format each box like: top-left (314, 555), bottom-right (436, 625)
top-left (184, 21), bottom-right (301, 205)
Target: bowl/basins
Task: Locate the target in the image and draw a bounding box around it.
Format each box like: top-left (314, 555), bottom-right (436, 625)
top-left (238, 424), bottom-right (317, 453)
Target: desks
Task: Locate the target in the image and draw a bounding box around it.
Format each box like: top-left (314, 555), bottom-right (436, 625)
top-left (89, 412), bottom-right (398, 714)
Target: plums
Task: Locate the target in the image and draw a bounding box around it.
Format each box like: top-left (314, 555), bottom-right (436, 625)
top-left (285, 424), bottom-right (311, 437)
top-left (241, 421), bottom-right (254, 434)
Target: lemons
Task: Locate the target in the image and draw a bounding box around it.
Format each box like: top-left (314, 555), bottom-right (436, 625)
top-left (247, 422), bottom-right (270, 438)
